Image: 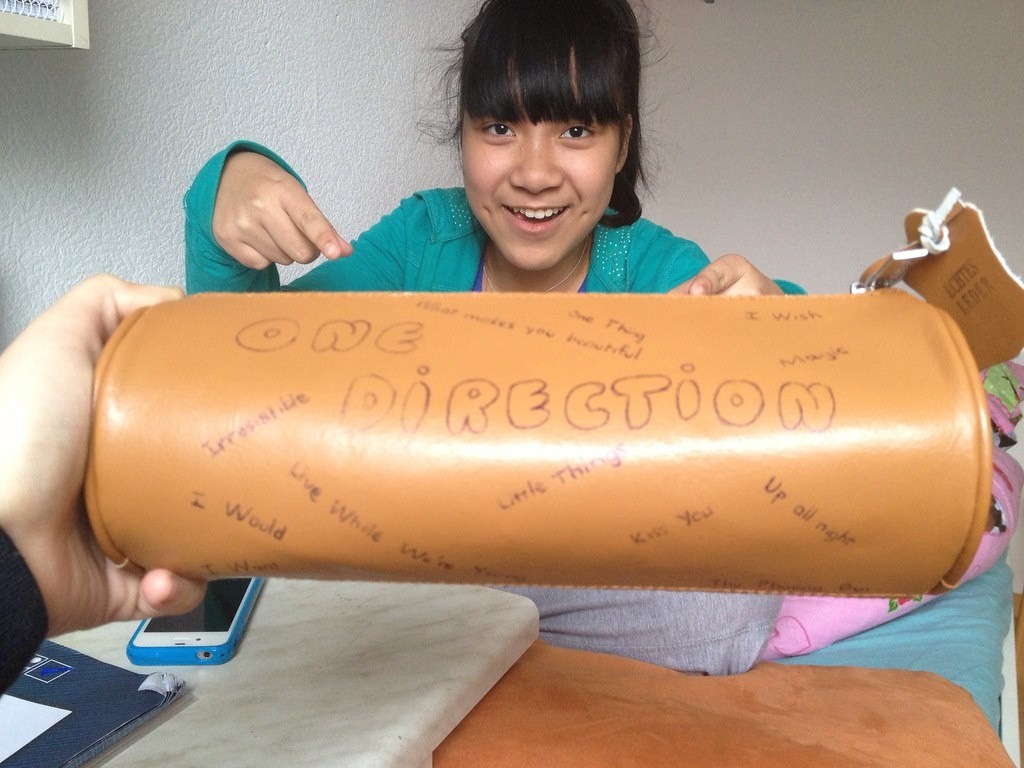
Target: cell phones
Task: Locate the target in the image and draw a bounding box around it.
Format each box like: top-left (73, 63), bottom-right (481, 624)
top-left (126, 574), bottom-right (265, 668)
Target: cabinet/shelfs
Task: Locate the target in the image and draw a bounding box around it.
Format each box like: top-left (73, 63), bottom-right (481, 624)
top-left (1, 0), bottom-right (91, 53)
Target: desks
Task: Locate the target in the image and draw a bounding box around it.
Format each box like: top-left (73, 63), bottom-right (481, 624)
top-left (45, 576), bottom-right (540, 768)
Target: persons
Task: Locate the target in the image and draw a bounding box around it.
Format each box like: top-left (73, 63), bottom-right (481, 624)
top-left (179, 0), bottom-right (816, 673)
top-left (0, 255), bottom-right (215, 711)
